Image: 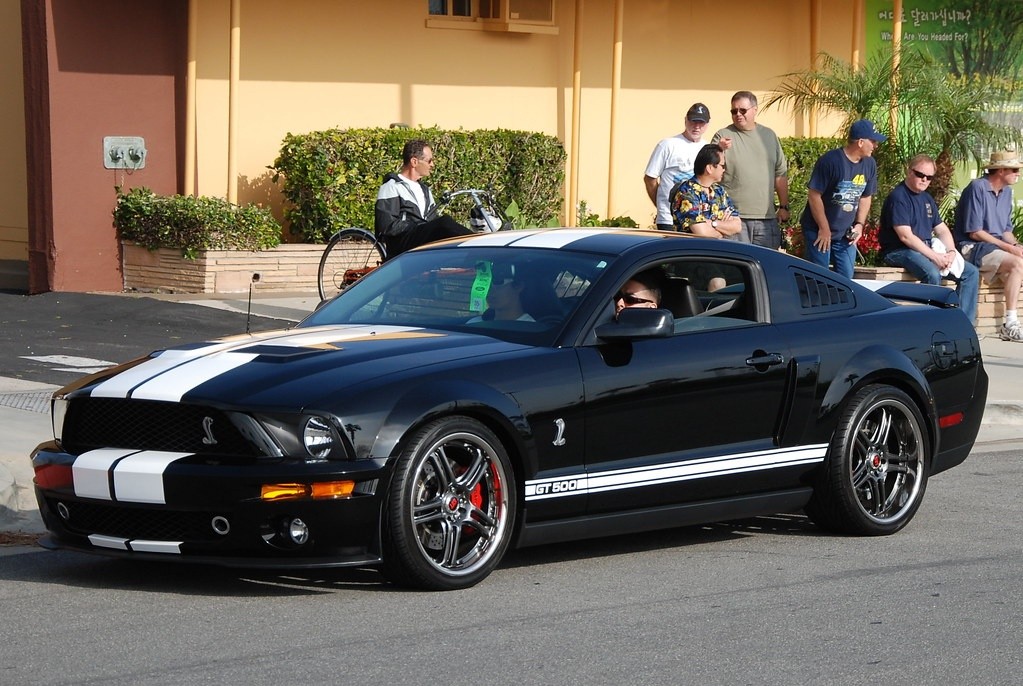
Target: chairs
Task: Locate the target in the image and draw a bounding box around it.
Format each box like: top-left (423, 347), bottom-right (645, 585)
top-left (660, 274), bottom-right (748, 319)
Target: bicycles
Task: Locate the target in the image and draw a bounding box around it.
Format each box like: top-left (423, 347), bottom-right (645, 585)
top-left (316, 188), bottom-right (507, 302)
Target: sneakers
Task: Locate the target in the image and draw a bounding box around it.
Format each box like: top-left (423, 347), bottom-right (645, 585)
top-left (999, 322), bottom-right (1023, 342)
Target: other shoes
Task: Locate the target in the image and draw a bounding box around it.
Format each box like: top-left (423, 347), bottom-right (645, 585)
top-left (976, 333), bottom-right (985, 340)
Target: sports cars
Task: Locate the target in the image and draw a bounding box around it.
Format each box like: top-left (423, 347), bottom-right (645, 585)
top-left (28, 226), bottom-right (990, 590)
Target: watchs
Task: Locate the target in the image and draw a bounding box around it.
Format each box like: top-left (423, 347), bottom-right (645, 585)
top-left (712, 220), bottom-right (719, 228)
top-left (780, 204), bottom-right (790, 212)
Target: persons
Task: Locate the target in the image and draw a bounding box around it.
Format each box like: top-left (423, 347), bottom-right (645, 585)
top-left (592, 263), bottom-right (672, 326)
top-left (466, 260), bottom-right (539, 322)
top-left (952, 150), bottom-right (1023, 343)
top-left (799, 119), bottom-right (886, 280)
top-left (373, 140), bottom-right (479, 262)
top-left (668, 144), bottom-right (741, 240)
top-left (878, 153), bottom-right (986, 342)
top-left (711, 90), bottom-right (790, 251)
top-left (643, 102), bottom-right (710, 231)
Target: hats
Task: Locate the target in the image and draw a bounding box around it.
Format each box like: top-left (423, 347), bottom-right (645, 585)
top-left (851, 120), bottom-right (887, 143)
top-left (687, 104), bottom-right (710, 123)
top-left (980, 151), bottom-right (1023, 169)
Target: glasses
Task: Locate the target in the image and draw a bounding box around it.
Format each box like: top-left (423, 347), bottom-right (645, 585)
top-left (418, 157), bottom-right (432, 164)
top-left (613, 290), bottom-right (653, 305)
top-left (999, 168), bottom-right (1018, 173)
top-left (705, 162), bottom-right (724, 169)
top-left (491, 278), bottom-right (514, 287)
top-left (909, 167), bottom-right (933, 180)
top-left (731, 105), bottom-right (753, 115)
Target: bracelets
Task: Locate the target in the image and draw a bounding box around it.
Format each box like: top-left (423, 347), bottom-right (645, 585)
top-left (1013, 242), bottom-right (1020, 246)
top-left (947, 250), bottom-right (956, 254)
top-left (853, 221), bottom-right (864, 227)
